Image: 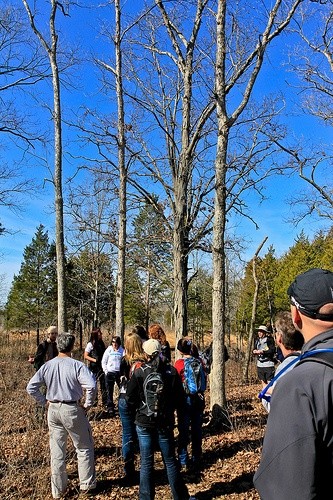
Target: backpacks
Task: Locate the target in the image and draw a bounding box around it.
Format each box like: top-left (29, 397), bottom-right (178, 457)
top-left (180, 356), bottom-right (205, 394)
top-left (136, 363), bottom-right (172, 418)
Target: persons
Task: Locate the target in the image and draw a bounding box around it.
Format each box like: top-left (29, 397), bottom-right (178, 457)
top-left (124, 325), bottom-right (210, 411)
top-left (254, 268), bottom-right (333, 500)
top-left (173, 340), bottom-right (207, 467)
top-left (34, 326), bottom-right (58, 394)
top-left (26, 332), bottom-right (97, 500)
top-left (252, 312), bottom-right (305, 414)
top-left (85, 327), bottom-right (106, 407)
top-left (101, 336), bottom-right (124, 413)
top-left (125, 339), bottom-right (193, 500)
top-left (118, 333), bottom-right (146, 474)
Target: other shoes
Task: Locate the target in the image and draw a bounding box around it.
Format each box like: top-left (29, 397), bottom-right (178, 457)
top-left (189, 496), bottom-right (198, 500)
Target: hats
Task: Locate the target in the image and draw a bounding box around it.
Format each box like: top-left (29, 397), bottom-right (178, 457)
top-left (142, 339), bottom-right (162, 355)
top-left (287, 268), bottom-right (333, 322)
top-left (255, 325), bottom-right (271, 332)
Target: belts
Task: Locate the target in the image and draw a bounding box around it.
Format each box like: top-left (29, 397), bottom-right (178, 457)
top-left (49, 399), bottom-right (81, 405)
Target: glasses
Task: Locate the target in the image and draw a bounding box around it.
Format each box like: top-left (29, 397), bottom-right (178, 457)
top-left (112, 341), bottom-right (117, 343)
top-left (274, 328), bottom-right (277, 333)
